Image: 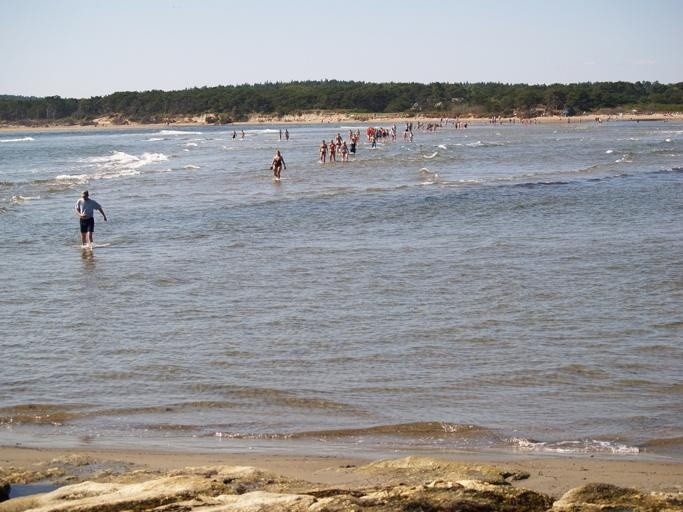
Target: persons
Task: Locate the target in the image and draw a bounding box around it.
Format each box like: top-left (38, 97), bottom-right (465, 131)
top-left (75, 190), bottom-right (107, 245)
top-left (319, 116), bottom-right (468, 164)
top-left (488, 109), bottom-right (681, 126)
top-left (232, 131), bottom-right (236, 141)
top-left (285, 128), bottom-right (289, 140)
top-left (241, 130), bottom-right (245, 138)
top-left (270, 151), bottom-right (286, 180)
